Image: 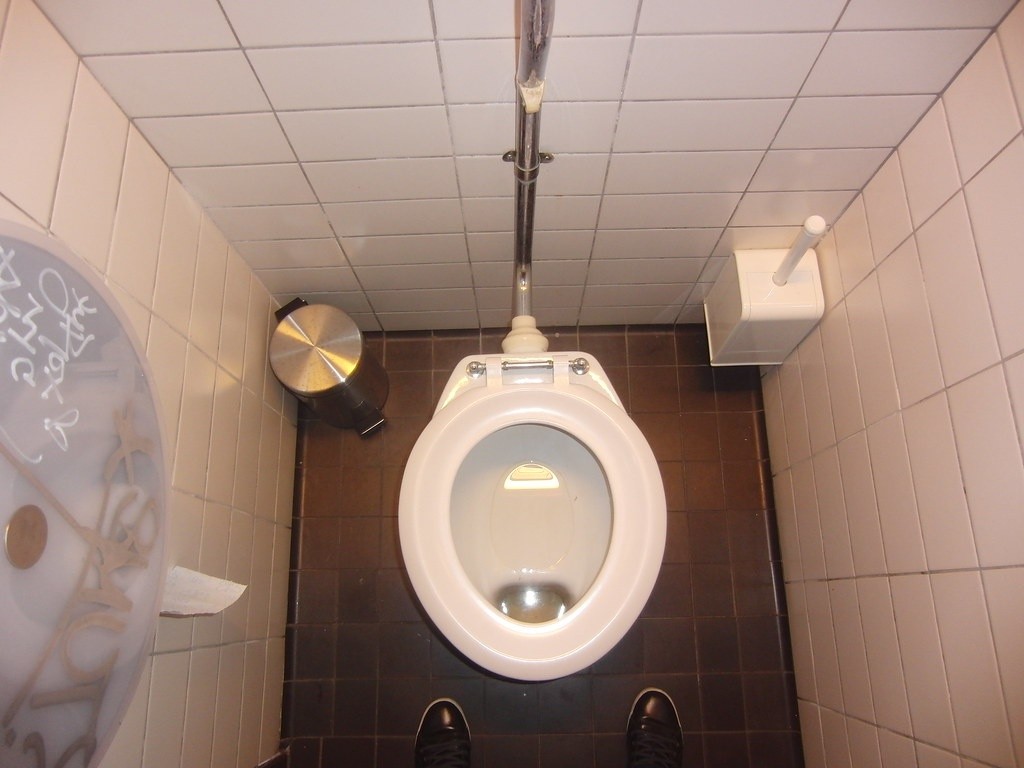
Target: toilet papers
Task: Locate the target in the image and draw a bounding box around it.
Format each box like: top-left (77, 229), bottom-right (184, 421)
top-left (0, 241), bottom-right (245, 767)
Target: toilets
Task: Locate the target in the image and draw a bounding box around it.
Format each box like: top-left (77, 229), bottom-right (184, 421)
top-left (397, 352), bottom-right (666, 682)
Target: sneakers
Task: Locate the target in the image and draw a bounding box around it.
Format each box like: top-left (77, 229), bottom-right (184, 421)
top-left (626, 687), bottom-right (684, 768)
top-left (414, 698), bottom-right (472, 768)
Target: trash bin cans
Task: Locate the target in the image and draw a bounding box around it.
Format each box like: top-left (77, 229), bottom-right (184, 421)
top-left (268, 297), bottom-right (390, 438)
